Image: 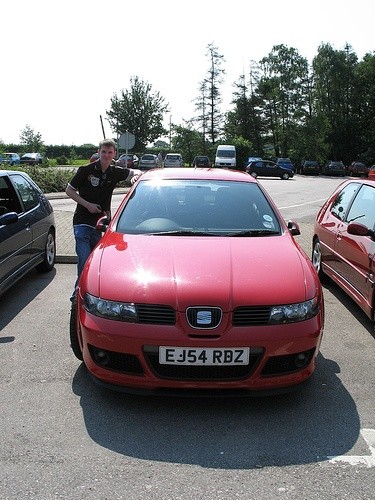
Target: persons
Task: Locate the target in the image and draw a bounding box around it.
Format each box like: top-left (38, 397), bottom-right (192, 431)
top-left (293, 161), bottom-right (301, 174)
top-left (155, 152), bottom-right (163, 168)
top-left (66, 139), bottom-right (144, 302)
top-left (188, 156), bottom-right (194, 167)
top-left (210, 153), bottom-right (215, 166)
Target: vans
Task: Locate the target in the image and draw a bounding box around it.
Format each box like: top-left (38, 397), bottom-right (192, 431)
top-left (163, 153), bottom-right (184, 168)
top-left (213, 145), bottom-right (237, 169)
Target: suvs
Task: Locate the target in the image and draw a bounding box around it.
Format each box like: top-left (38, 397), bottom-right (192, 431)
top-left (68, 167), bottom-right (325, 400)
top-left (295, 160), bottom-right (320, 176)
top-left (191, 156), bottom-right (213, 168)
top-left (275, 158), bottom-right (293, 170)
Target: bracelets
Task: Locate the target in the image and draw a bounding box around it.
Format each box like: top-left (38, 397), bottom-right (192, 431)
top-left (134, 174), bottom-right (140, 177)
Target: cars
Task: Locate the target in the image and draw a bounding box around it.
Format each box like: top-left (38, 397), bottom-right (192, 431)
top-left (245, 160), bottom-right (295, 180)
top-left (367, 164), bottom-right (375, 181)
top-left (310, 178), bottom-right (375, 327)
top-left (346, 161), bottom-right (369, 177)
top-left (0, 153), bottom-right (21, 167)
top-left (19, 153), bottom-right (49, 166)
top-left (90, 152), bottom-right (139, 169)
top-left (322, 160), bottom-right (347, 177)
top-left (138, 154), bottom-right (161, 170)
top-left (0, 169), bottom-right (57, 300)
top-left (247, 157), bottom-right (263, 168)
